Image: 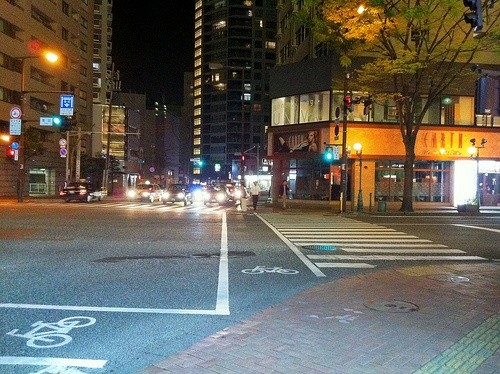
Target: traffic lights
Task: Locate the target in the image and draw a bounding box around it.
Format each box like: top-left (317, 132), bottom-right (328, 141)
top-left (1, 134), bottom-right (13, 145)
top-left (324, 147), bottom-right (333, 161)
top-left (462, 0), bottom-right (483, 32)
top-left (52, 116), bottom-right (63, 126)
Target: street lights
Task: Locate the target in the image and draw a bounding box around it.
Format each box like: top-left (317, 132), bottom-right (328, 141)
top-left (468, 138), bottom-right (487, 210)
top-left (354, 143), bottom-right (365, 210)
top-left (16, 53), bottom-right (57, 203)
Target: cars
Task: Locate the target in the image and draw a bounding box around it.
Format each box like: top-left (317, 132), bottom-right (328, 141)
top-left (59, 182), bottom-right (101, 203)
top-left (128, 183), bottom-right (243, 206)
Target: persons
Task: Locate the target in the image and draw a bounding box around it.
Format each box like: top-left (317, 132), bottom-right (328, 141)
top-left (251, 181), bottom-right (260, 210)
top-left (279, 131), bottom-right (317, 152)
top-left (241, 184), bottom-right (248, 211)
top-left (279, 180), bottom-right (290, 208)
